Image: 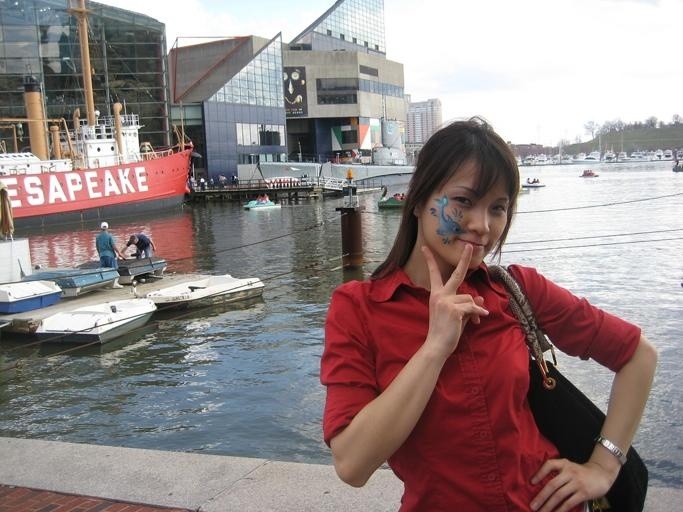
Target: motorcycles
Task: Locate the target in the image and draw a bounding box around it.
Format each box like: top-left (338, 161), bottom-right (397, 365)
top-left (242, 199), bottom-right (281, 211)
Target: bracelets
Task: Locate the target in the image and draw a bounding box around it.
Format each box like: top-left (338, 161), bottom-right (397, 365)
top-left (594, 435), bottom-right (628, 465)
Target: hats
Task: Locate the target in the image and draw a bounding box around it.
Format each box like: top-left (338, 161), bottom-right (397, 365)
top-left (100, 222), bottom-right (108, 229)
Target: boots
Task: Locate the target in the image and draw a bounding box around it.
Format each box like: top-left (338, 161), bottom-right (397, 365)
top-left (112, 277), bottom-right (122, 288)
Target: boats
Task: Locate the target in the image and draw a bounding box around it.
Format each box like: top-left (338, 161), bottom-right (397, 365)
top-left (1, 256), bottom-right (265, 344)
top-left (522, 183), bottom-right (546, 187)
top-left (579, 175), bottom-right (600, 177)
top-left (378, 185), bottom-right (409, 209)
top-left (514, 135), bottom-right (683, 165)
top-left (672, 160), bottom-right (683, 172)
top-left (0, 0), bottom-right (194, 229)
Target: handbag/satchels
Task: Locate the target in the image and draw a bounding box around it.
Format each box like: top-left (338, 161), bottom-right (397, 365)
top-left (488, 263), bottom-right (649, 511)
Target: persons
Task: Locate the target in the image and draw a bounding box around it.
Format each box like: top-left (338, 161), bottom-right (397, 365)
top-left (393, 193), bottom-right (408, 201)
top-left (256, 193), bottom-right (269, 203)
top-left (96, 221), bottom-right (156, 289)
top-left (189, 173), bottom-right (238, 191)
top-left (582, 169), bottom-right (594, 177)
top-left (527, 177), bottom-right (540, 184)
top-left (318, 117), bottom-right (657, 512)
top-left (94, 105), bottom-right (100, 125)
